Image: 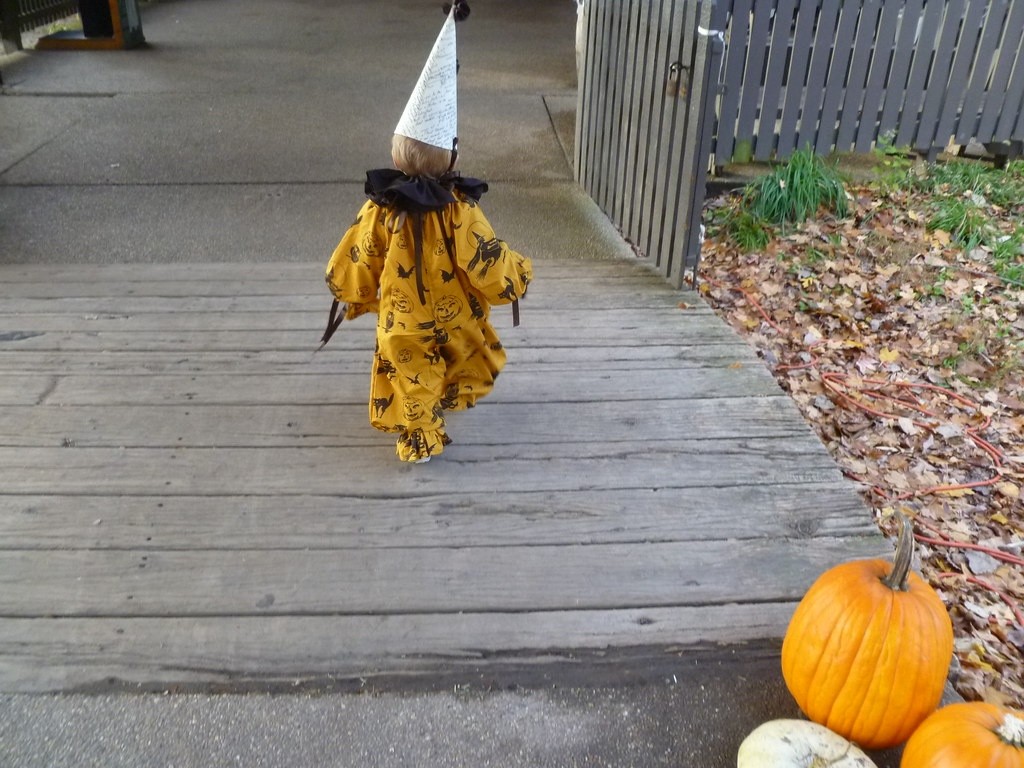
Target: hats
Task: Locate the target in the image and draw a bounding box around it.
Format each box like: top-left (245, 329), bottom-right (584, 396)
top-left (394, 0), bottom-right (470, 151)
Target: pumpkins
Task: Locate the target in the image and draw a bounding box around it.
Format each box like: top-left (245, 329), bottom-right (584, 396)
top-left (733, 512), bottom-right (1024, 768)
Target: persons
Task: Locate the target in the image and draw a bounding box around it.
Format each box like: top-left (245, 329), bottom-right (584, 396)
top-left (324, 6), bottom-right (534, 463)
